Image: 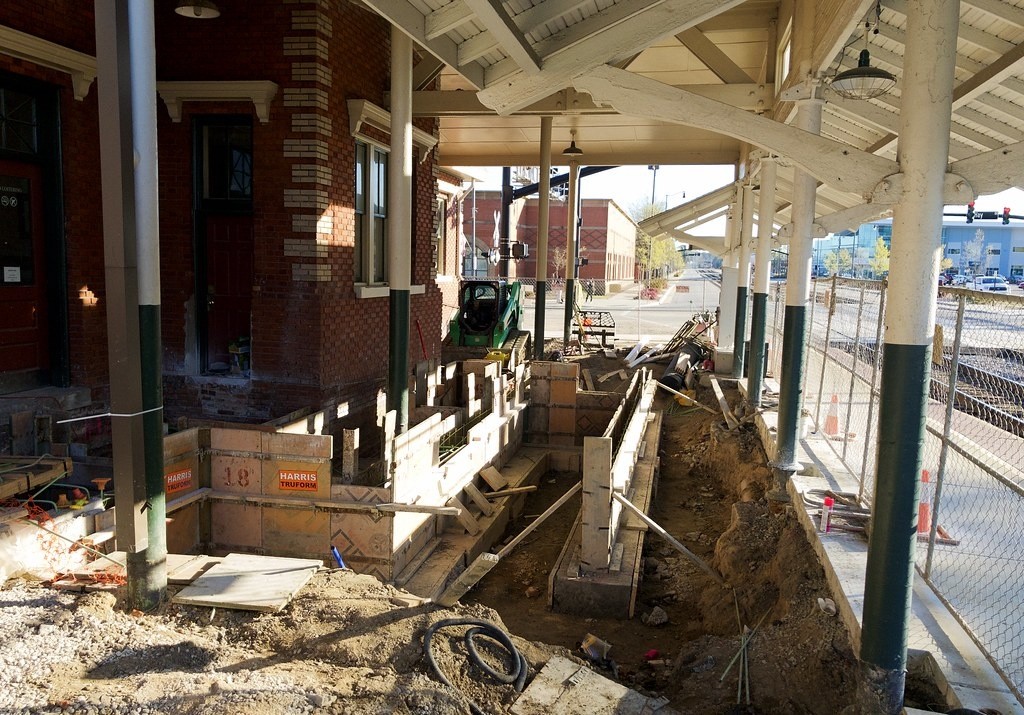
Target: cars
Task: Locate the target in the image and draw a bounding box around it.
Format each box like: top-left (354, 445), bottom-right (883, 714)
top-left (939, 273), bottom-right (1007, 286)
top-left (1007, 275), bottom-right (1023, 284)
top-left (973, 276), bottom-right (1010, 295)
top-left (1019, 282), bottom-right (1024, 290)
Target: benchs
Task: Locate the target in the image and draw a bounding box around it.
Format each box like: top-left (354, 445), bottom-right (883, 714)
top-left (572, 311), bottom-right (615, 347)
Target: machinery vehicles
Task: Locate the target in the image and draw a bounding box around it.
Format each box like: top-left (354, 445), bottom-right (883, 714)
top-left (440, 280), bottom-right (534, 368)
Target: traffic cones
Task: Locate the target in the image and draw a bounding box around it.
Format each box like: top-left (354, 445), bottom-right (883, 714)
top-left (822, 394), bottom-right (846, 442)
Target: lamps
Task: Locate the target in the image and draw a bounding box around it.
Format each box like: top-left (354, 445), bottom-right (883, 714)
top-left (562, 132), bottom-right (584, 158)
top-left (830, 25), bottom-right (895, 101)
top-left (174, 0), bottom-right (220, 19)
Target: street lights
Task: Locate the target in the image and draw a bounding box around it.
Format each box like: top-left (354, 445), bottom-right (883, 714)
top-left (665, 190), bottom-right (686, 209)
top-left (647, 165), bottom-right (659, 289)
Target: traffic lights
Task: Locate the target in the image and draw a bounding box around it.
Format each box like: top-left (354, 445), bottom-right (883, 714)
top-left (966, 202), bottom-right (974, 224)
top-left (1003, 207), bottom-right (1010, 225)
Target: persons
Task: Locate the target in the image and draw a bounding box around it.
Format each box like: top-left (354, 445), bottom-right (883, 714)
top-left (495, 274), bottom-right (501, 281)
top-left (556, 277), bottom-right (564, 304)
top-left (585, 277), bottom-right (595, 303)
